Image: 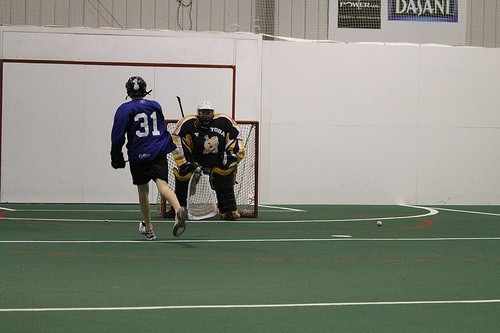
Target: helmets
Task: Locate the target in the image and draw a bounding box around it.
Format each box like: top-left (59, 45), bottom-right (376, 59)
top-left (197, 101), bottom-right (214, 111)
top-left (125, 77), bottom-right (148, 97)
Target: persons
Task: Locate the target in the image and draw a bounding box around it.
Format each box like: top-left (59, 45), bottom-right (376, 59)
top-left (110, 75), bottom-right (189, 242)
top-left (165, 101), bottom-right (246, 220)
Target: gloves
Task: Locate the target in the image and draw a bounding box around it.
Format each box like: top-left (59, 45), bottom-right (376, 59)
top-left (110, 143), bottom-right (125, 169)
top-left (173, 162), bottom-right (201, 183)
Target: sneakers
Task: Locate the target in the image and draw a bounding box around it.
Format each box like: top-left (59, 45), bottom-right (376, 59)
top-left (138, 220), bottom-right (156, 240)
top-left (163, 209), bottom-right (175, 219)
top-left (173, 207), bottom-right (188, 237)
top-left (223, 210), bottom-right (237, 221)
